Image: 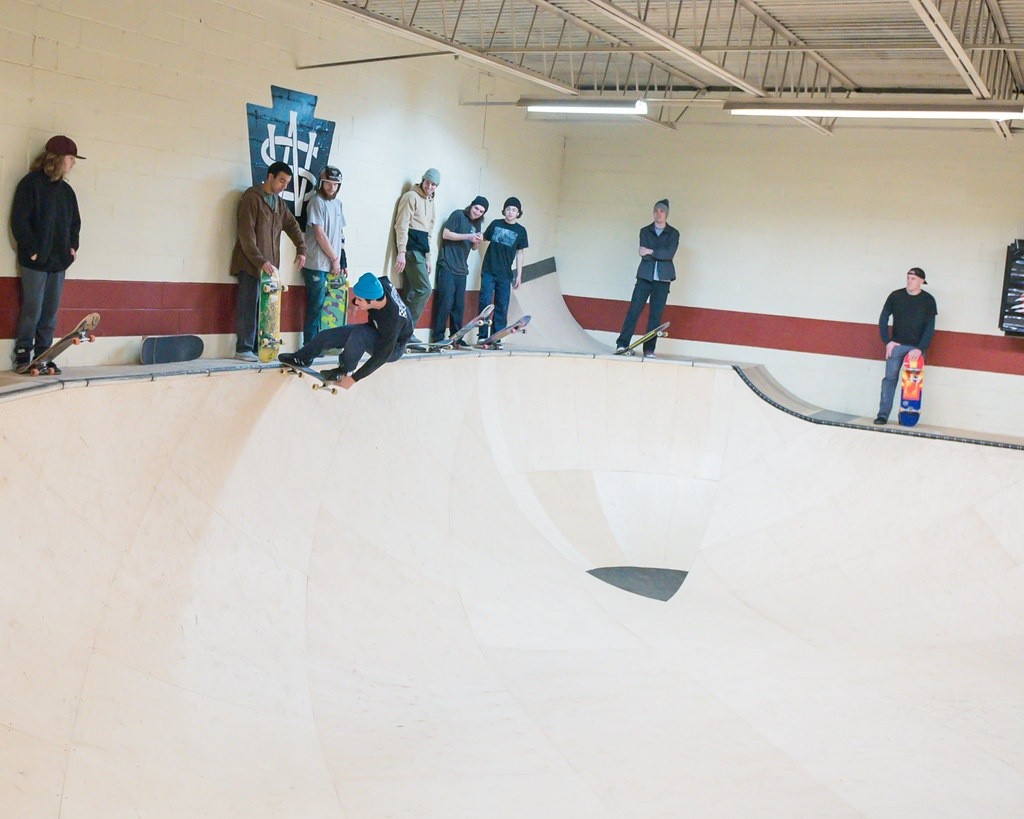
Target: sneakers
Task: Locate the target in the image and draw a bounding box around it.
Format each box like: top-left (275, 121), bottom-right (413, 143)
top-left (234, 349), bottom-right (259, 362)
top-left (320, 367), bottom-right (348, 382)
top-left (874, 417), bottom-right (886, 424)
top-left (476, 333), bottom-right (488, 344)
top-left (33, 353), bottom-right (61, 375)
top-left (456, 340), bottom-right (474, 350)
top-left (643, 350), bottom-right (656, 358)
top-left (14, 345), bottom-right (31, 374)
top-left (616, 342), bottom-right (626, 351)
top-left (278, 352), bottom-right (314, 368)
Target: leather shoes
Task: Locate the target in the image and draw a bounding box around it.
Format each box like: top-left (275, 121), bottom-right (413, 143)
top-left (406, 334), bottom-right (422, 343)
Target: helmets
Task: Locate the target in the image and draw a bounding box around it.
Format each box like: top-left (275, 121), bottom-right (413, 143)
top-left (316, 165), bottom-right (342, 189)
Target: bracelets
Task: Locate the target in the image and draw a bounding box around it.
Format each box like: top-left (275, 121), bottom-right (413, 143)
top-left (331, 256), bottom-right (339, 264)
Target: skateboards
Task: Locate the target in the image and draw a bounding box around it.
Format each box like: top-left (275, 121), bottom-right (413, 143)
top-left (480, 314), bottom-right (531, 349)
top-left (258, 263), bottom-right (288, 365)
top-left (450, 304), bottom-right (494, 348)
top-left (320, 271), bottom-right (349, 356)
top-left (898, 352), bottom-right (925, 427)
top-left (17, 312), bottom-right (100, 376)
top-left (614, 321), bottom-right (671, 356)
top-left (139, 334), bottom-right (204, 365)
top-left (405, 339), bottom-right (453, 354)
top-left (277, 351), bottom-right (343, 395)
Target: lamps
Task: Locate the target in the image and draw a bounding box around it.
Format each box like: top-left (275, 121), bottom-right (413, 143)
top-left (515, 98), bottom-right (648, 114)
top-left (721, 105), bottom-right (1024, 119)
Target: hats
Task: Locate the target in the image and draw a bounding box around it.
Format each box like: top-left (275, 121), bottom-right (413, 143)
top-left (504, 197), bottom-right (522, 213)
top-left (45, 136), bottom-right (87, 160)
top-left (470, 196), bottom-right (489, 214)
top-left (655, 199), bottom-right (669, 215)
top-left (422, 168), bottom-right (440, 187)
top-left (907, 267), bottom-right (928, 285)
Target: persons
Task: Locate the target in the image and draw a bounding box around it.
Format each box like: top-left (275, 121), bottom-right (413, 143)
top-left (275, 272), bottom-right (416, 390)
top-left (431, 194), bottom-right (489, 350)
top-left (10, 133), bottom-right (89, 373)
top-left (618, 197), bottom-right (682, 359)
top-left (475, 195), bottom-right (528, 351)
top-left (226, 161), bottom-right (310, 363)
top-left (393, 166), bottom-right (442, 352)
top-left (873, 266), bottom-right (938, 428)
top-left (295, 165), bottom-right (348, 358)
